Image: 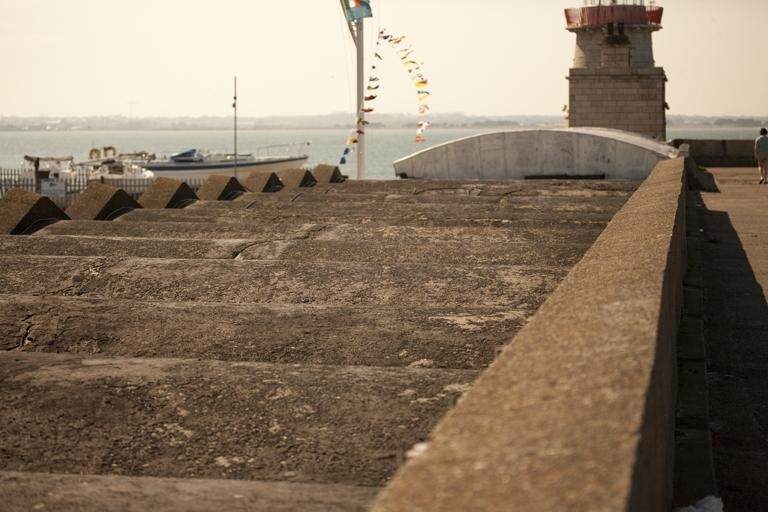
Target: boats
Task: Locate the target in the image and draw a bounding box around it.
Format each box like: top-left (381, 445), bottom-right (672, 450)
top-left (71, 148), bottom-right (310, 193)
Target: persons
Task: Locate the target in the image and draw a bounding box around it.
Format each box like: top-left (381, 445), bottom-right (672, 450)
top-left (754, 127), bottom-right (768, 184)
